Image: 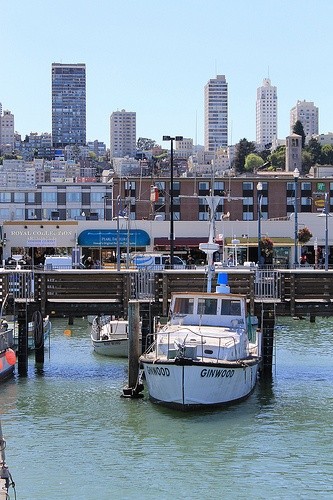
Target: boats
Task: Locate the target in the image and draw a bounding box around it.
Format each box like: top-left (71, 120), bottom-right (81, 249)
top-left (90, 315), bottom-right (146, 357)
top-left (0, 326), bottom-right (16, 384)
top-left (12, 315), bottom-right (52, 352)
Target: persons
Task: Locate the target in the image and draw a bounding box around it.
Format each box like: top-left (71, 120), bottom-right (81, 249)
top-left (258, 255), bottom-right (265, 264)
top-left (84, 257), bottom-right (98, 268)
top-left (5, 257), bottom-right (30, 269)
top-left (165, 257), bottom-right (170, 268)
top-left (228, 254), bottom-right (235, 265)
top-left (301, 255), bottom-right (307, 267)
top-left (187, 255), bottom-right (195, 269)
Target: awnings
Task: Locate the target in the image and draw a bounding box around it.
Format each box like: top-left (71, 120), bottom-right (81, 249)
top-left (225, 237), bottom-right (295, 246)
top-left (78, 229), bottom-right (149, 246)
top-left (154, 237), bottom-right (223, 245)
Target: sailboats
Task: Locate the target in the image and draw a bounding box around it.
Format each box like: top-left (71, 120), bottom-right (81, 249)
top-left (139, 158), bottom-right (262, 412)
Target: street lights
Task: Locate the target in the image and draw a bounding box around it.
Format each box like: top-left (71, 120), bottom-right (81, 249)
top-left (163, 135), bottom-right (184, 269)
top-left (292, 167), bottom-right (300, 269)
top-left (256, 181), bottom-right (263, 269)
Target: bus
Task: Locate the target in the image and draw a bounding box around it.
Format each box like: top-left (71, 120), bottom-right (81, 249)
top-left (223, 242), bottom-right (333, 269)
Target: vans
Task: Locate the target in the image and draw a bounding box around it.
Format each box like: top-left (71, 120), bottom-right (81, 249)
top-left (134, 254), bottom-right (187, 269)
